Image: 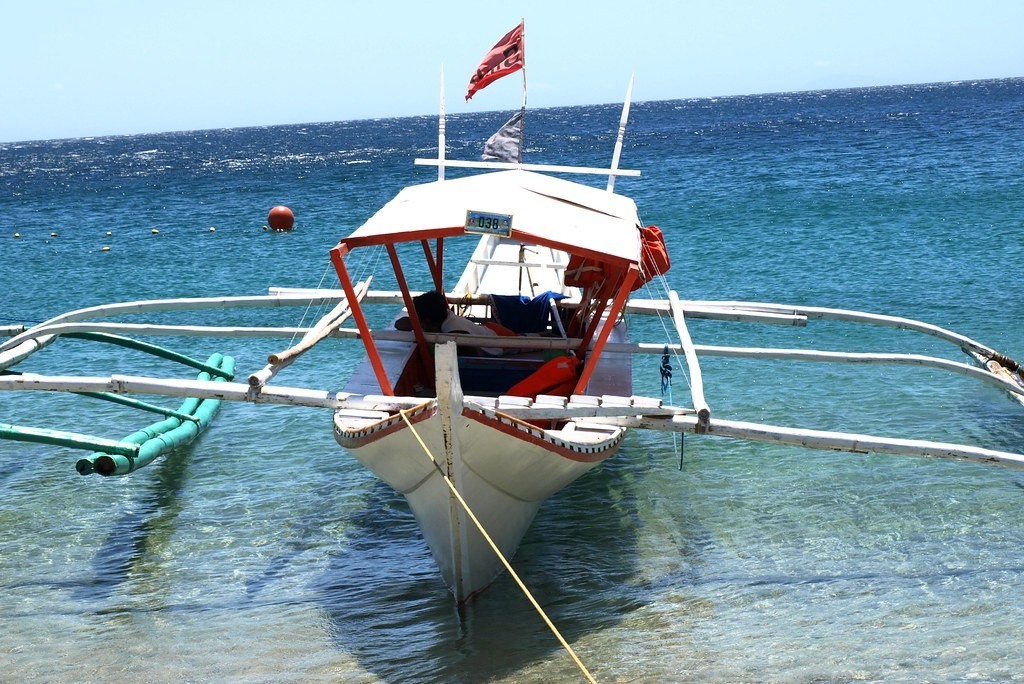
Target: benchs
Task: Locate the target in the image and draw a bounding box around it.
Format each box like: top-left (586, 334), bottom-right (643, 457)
top-left (439, 328), bottom-right (567, 363)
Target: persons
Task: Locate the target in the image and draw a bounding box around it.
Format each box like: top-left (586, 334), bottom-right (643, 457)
top-left (500, 219), bottom-right (509, 230)
top-left (396, 289), bottom-right (558, 356)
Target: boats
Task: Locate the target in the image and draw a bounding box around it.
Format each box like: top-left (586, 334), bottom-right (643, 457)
top-left (0, 19), bottom-right (1024, 605)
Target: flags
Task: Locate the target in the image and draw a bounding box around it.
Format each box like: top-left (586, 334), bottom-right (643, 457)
top-left (465, 21), bottom-right (525, 101)
top-left (481, 111), bottom-right (520, 164)
